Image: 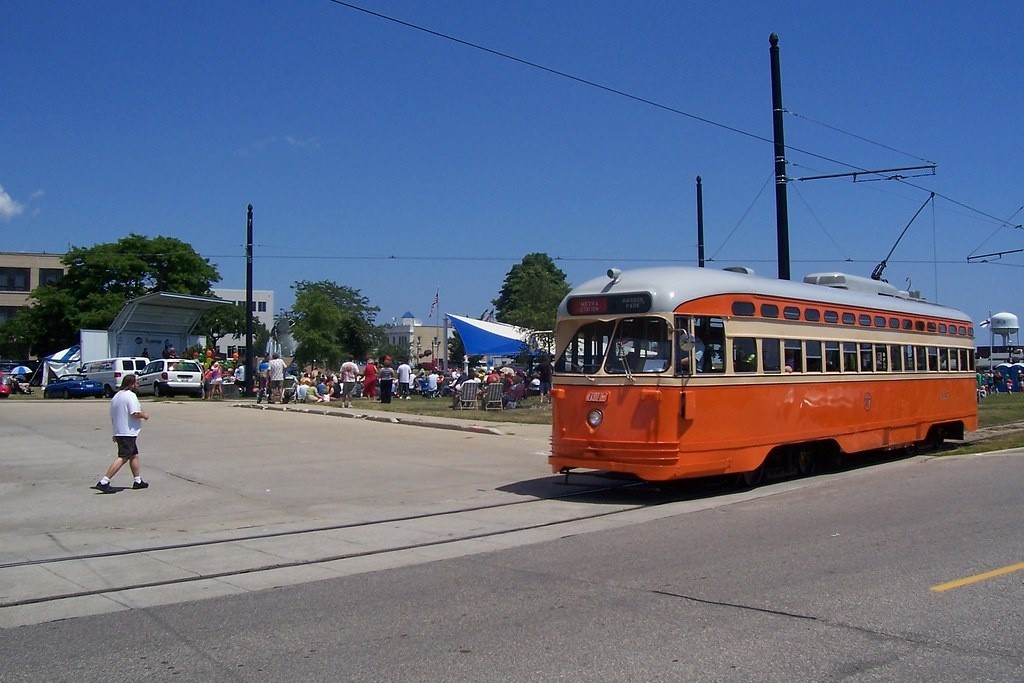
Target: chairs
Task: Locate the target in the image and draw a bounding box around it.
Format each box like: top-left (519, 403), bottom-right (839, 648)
top-left (528, 383), bottom-right (540, 400)
top-left (483, 383), bottom-right (504, 412)
top-left (459, 383), bottom-right (478, 411)
top-left (68, 378), bottom-right (74, 381)
top-left (508, 384), bottom-right (524, 409)
top-left (283, 377), bottom-right (297, 404)
top-left (83, 378), bottom-right (89, 380)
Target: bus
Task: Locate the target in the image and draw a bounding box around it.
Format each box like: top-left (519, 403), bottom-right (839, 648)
top-left (548, 266), bottom-right (979, 484)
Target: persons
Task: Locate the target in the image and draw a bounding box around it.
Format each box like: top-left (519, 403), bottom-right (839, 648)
top-left (162, 344), bottom-right (175, 359)
top-left (96, 374), bottom-right (149, 494)
top-left (359, 359), bottom-right (379, 402)
top-left (785, 350), bottom-right (799, 373)
top-left (413, 363), bottom-right (425, 388)
top-left (534, 356), bottom-right (554, 404)
top-left (431, 367), bottom-right (536, 410)
top-left (142, 348), bottom-right (149, 359)
top-left (12, 375), bottom-right (19, 383)
top-left (204, 353), bottom-right (341, 404)
top-left (409, 368), bottom-right (416, 393)
top-left (397, 360), bottom-right (412, 400)
top-left (658, 318), bottom-right (706, 373)
top-left (378, 358), bottom-right (395, 403)
top-left (339, 355), bottom-right (359, 408)
top-left (427, 369), bottom-right (438, 399)
top-left (985, 370), bottom-right (1024, 395)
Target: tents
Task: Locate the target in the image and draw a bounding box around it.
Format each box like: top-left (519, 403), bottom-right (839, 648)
top-left (28, 344), bottom-right (80, 391)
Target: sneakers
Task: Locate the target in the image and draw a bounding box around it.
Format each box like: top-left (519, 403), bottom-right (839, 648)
top-left (95, 482), bottom-right (116, 494)
top-left (133, 479), bottom-right (149, 489)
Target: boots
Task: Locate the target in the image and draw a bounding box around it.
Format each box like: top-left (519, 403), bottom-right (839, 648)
top-left (348, 402), bottom-right (353, 408)
top-left (341, 402), bottom-right (345, 408)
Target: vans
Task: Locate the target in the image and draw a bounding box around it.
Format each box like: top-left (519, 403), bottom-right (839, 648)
top-left (77, 356), bottom-right (151, 398)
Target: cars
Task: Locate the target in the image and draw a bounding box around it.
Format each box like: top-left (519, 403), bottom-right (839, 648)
top-left (134, 359), bottom-right (205, 398)
top-left (44, 374), bottom-right (104, 399)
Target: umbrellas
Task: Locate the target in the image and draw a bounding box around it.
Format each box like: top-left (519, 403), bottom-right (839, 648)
top-left (10, 366), bottom-right (33, 374)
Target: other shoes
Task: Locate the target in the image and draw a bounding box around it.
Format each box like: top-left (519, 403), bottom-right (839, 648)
top-left (399, 397), bottom-right (402, 399)
top-left (406, 396), bottom-right (411, 400)
